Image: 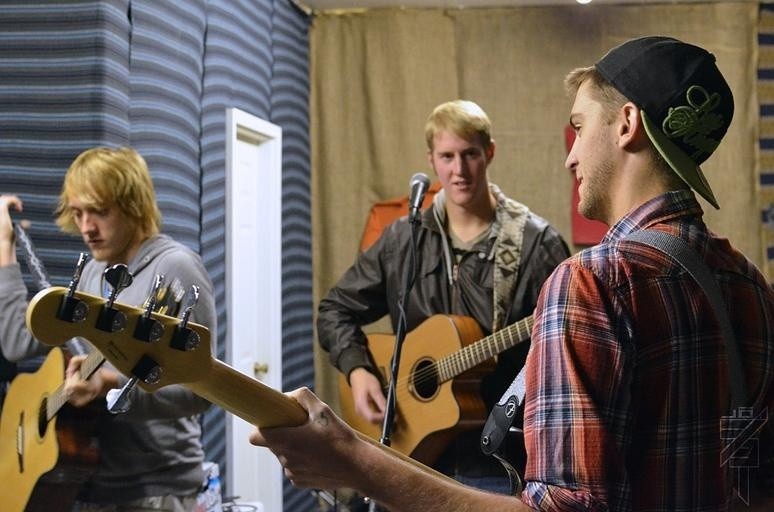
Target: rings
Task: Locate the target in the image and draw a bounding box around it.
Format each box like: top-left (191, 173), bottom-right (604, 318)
top-left (66, 391), bottom-right (74, 400)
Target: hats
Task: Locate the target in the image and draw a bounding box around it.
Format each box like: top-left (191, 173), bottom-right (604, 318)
top-left (592, 32), bottom-right (734, 210)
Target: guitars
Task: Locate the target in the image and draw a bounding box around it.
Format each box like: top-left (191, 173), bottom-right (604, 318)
top-left (338, 312), bottom-right (534, 462)
top-left (26, 251), bottom-right (466, 512)
top-left (0, 280), bottom-right (192, 512)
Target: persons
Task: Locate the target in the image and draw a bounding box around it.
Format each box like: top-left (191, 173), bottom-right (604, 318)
top-left (316, 99), bottom-right (571, 495)
top-left (248, 33), bottom-right (774, 512)
top-left (0, 142), bottom-right (218, 512)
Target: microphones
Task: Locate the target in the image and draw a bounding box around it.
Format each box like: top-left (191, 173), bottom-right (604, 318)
top-left (406, 171), bottom-right (432, 221)
